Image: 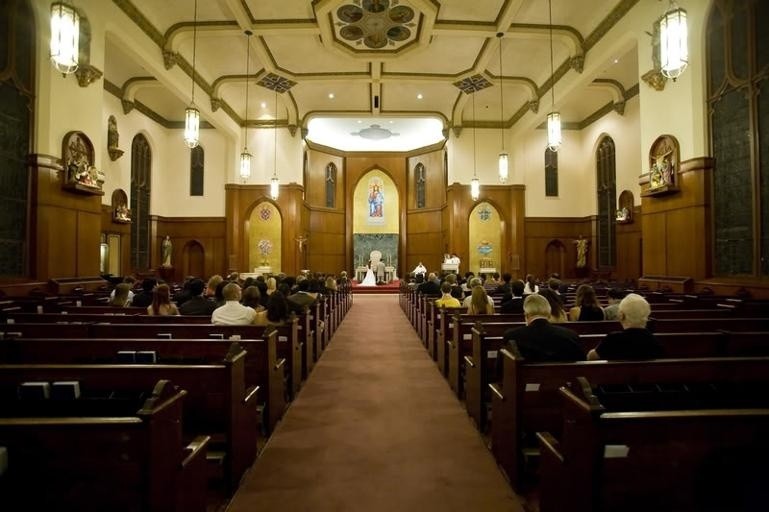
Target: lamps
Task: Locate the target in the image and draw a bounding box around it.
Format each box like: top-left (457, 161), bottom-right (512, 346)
top-left (236, 29), bottom-right (255, 186)
top-left (656, 1), bottom-right (691, 84)
top-left (180, 1), bottom-right (203, 146)
top-left (50, 1), bottom-right (80, 78)
top-left (467, 87), bottom-right (483, 204)
top-left (269, 86), bottom-right (282, 203)
top-left (544, 1), bottom-right (566, 151)
top-left (492, 32), bottom-right (511, 186)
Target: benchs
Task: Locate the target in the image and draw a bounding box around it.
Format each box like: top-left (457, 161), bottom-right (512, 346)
top-left (0, 279), bottom-right (358, 512)
top-left (400, 278), bottom-right (768, 510)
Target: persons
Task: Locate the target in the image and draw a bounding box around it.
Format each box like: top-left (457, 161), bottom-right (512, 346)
top-left (368, 184), bottom-right (384, 217)
top-left (407, 252), bottom-right (665, 391)
top-left (106, 268), bottom-right (353, 327)
top-left (162, 234), bottom-right (173, 269)
top-left (355, 259), bottom-right (376, 287)
top-left (376, 258), bottom-right (386, 285)
top-left (575, 233), bottom-right (588, 268)
